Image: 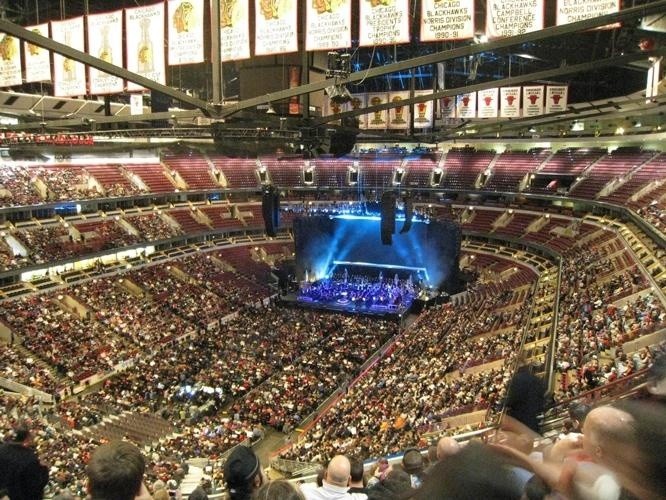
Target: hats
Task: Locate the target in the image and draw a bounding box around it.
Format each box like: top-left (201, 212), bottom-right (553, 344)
top-left (224, 446), bottom-right (258, 486)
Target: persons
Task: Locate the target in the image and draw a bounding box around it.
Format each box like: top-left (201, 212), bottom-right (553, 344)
top-left (3, 164), bottom-right (666, 499)
